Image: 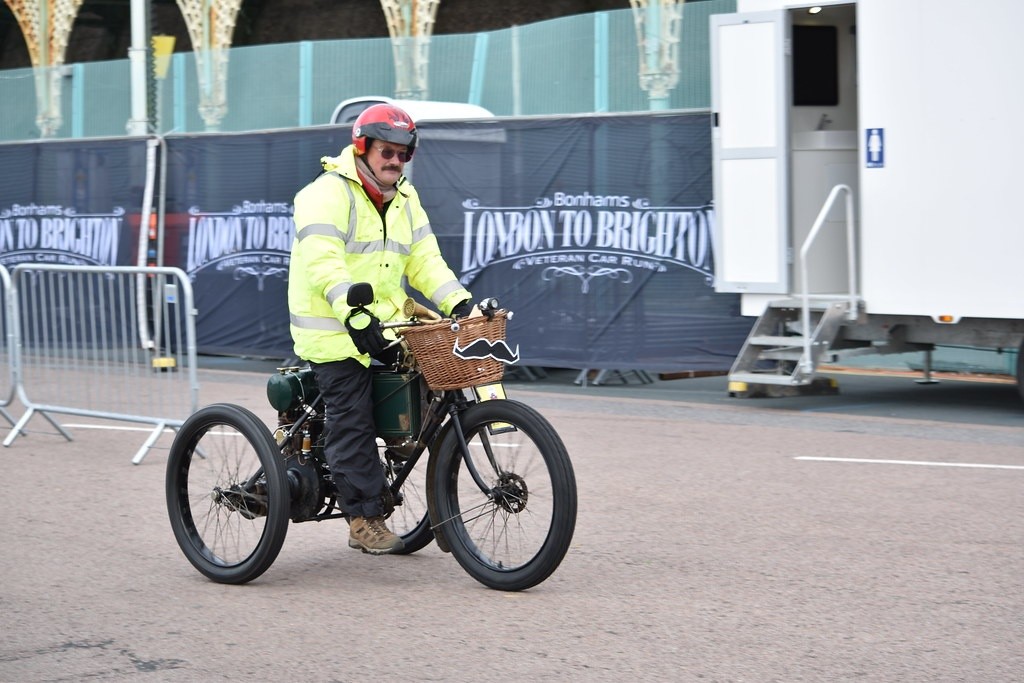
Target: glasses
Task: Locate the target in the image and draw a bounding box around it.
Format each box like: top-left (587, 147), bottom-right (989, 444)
top-left (371, 143), bottom-right (408, 163)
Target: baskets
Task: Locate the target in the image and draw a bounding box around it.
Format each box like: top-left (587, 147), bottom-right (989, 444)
top-left (396, 308), bottom-right (520, 391)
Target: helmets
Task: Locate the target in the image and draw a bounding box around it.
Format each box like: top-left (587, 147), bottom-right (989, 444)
top-left (352, 104), bottom-right (419, 162)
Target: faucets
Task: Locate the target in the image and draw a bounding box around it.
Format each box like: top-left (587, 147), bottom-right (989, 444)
top-left (816, 114), bottom-right (833, 131)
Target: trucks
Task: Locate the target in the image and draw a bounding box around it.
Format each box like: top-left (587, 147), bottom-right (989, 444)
top-left (709, 0), bottom-right (1024, 407)
top-left (327, 96), bottom-right (507, 237)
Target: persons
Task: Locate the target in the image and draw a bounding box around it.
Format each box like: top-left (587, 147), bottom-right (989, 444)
top-left (288, 104), bottom-right (471, 555)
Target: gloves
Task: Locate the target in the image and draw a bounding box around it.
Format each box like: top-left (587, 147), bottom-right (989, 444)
top-left (344, 307), bottom-right (388, 356)
top-left (449, 299), bottom-right (496, 321)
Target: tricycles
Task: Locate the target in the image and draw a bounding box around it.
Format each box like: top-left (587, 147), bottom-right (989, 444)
top-left (165, 282), bottom-right (578, 592)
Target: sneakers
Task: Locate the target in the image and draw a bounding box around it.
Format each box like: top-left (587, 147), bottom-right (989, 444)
top-left (383, 436), bottom-right (416, 458)
top-left (348, 516), bottom-right (405, 555)
